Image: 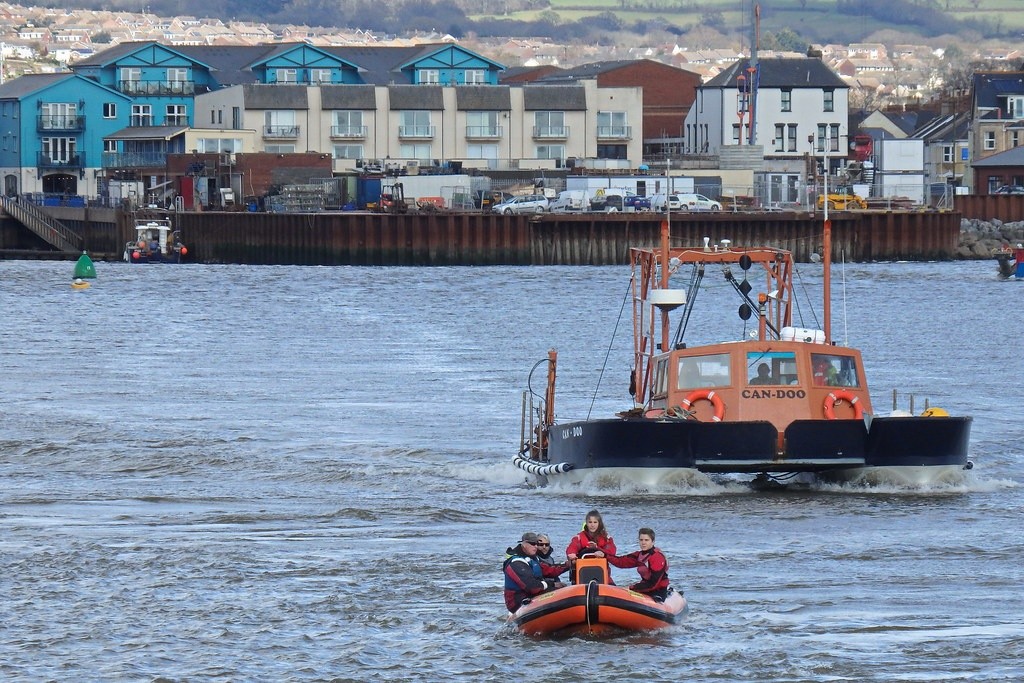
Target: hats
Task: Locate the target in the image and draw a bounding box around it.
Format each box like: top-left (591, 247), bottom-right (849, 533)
top-left (518, 533), bottom-right (538, 543)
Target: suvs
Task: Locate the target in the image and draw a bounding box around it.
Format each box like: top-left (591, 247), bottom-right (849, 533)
top-left (591, 195), bottom-right (624, 211)
top-left (623, 196), bottom-right (651, 209)
top-left (492, 194), bottom-right (547, 215)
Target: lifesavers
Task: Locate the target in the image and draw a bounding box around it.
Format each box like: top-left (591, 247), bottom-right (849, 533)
top-left (680, 389), bottom-right (725, 424)
top-left (822, 389), bottom-right (865, 420)
top-left (72, 282), bottom-right (90, 289)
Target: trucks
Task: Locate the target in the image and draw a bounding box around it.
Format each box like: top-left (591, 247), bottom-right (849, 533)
top-left (603, 188), bottom-right (637, 197)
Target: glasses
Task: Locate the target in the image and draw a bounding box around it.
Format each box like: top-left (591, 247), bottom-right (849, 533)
top-left (526, 541), bottom-right (538, 546)
top-left (538, 543), bottom-right (550, 546)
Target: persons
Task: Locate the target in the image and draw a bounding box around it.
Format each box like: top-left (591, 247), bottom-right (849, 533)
top-left (595, 528), bottom-right (669, 602)
top-left (566, 509), bottom-right (617, 585)
top-left (812, 356), bottom-right (839, 386)
top-left (65, 186), bottom-right (74, 207)
top-left (750, 363), bottom-right (778, 385)
top-left (536, 533), bottom-right (560, 583)
top-left (503, 533), bottom-right (575, 613)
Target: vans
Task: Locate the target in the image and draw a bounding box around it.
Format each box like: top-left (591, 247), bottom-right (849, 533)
top-left (650, 193), bottom-right (681, 210)
top-left (548, 190), bottom-right (591, 212)
top-left (677, 194), bottom-right (723, 211)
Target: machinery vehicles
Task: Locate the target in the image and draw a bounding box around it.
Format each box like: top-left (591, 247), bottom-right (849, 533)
top-left (371, 183), bottom-right (407, 213)
top-left (816, 193), bottom-right (868, 211)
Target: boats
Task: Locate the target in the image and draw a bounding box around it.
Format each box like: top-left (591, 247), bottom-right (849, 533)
top-left (125, 218), bottom-right (185, 265)
top-left (515, 554), bottom-right (676, 638)
top-left (995, 244), bottom-right (1024, 278)
top-left (515, 138), bottom-right (978, 498)
